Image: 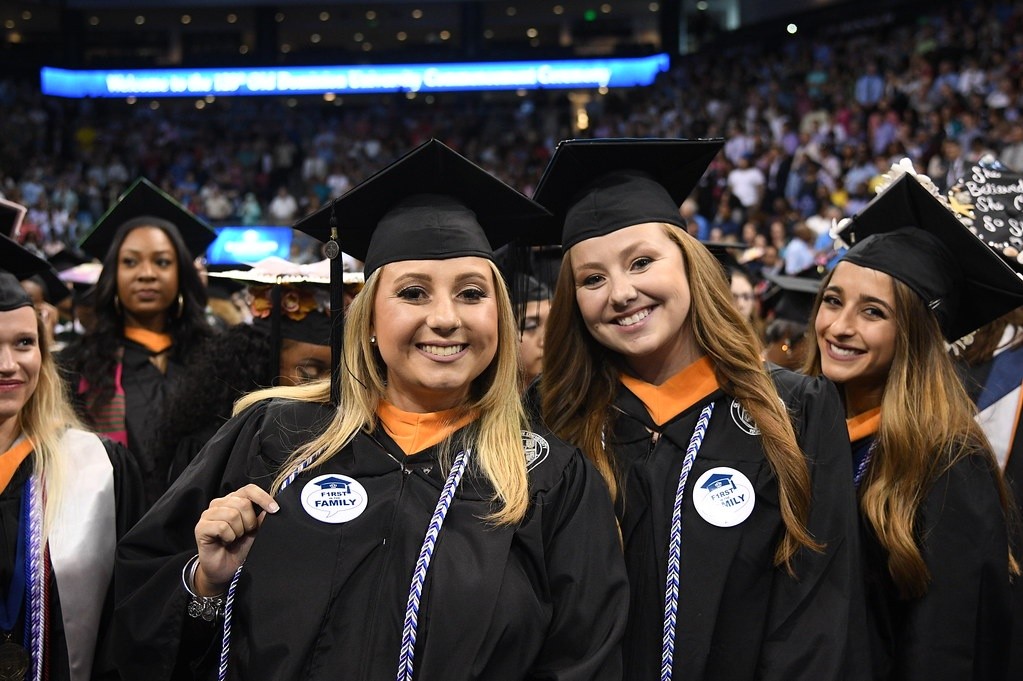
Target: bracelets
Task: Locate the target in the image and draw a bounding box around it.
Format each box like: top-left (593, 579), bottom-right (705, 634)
top-left (183, 552), bottom-right (226, 621)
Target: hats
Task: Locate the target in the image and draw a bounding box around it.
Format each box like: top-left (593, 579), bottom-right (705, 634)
top-left (835, 172), bottom-right (1023, 345)
top-left (77, 177), bottom-right (218, 266)
top-left (207, 134), bottom-right (563, 407)
top-left (0, 232), bottom-right (107, 315)
top-left (701, 241), bottom-right (826, 323)
top-left (522, 136), bottom-right (727, 257)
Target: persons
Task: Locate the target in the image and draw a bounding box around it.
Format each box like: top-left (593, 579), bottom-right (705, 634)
top-left (534, 134), bottom-right (851, 681)
top-left (0, 233), bottom-right (138, 679)
top-left (64, 180), bottom-right (228, 525)
top-left (804, 175), bottom-right (1023, 680)
top-left (98, 141), bottom-right (631, 681)
top-left (0, 0), bottom-right (1023, 587)
